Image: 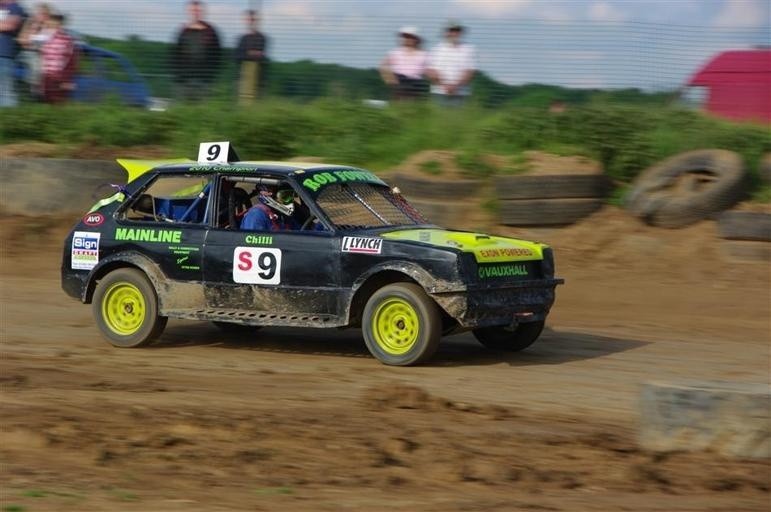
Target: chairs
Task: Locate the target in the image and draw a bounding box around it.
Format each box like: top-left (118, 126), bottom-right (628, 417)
top-left (228, 188), bottom-right (252, 231)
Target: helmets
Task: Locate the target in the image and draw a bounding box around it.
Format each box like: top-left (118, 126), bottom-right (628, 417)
top-left (255, 183), bottom-right (296, 216)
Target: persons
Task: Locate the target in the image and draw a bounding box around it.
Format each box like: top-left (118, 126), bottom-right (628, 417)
top-left (164, 0), bottom-right (224, 102)
top-left (235, 10), bottom-right (271, 98)
top-left (426, 19), bottom-right (478, 101)
top-left (380, 26), bottom-right (429, 103)
top-left (239, 179), bottom-right (322, 232)
top-left (1, 0), bottom-right (80, 109)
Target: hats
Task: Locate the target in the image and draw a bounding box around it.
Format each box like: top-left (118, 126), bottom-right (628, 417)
top-left (437, 19), bottom-right (463, 31)
top-left (396, 26), bottom-right (421, 41)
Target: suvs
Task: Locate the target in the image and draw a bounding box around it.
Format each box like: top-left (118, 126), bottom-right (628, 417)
top-left (61, 158), bottom-right (564, 368)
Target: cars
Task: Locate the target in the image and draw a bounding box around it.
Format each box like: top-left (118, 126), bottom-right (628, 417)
top-left (14, 41), bottom-right (150, 111)
